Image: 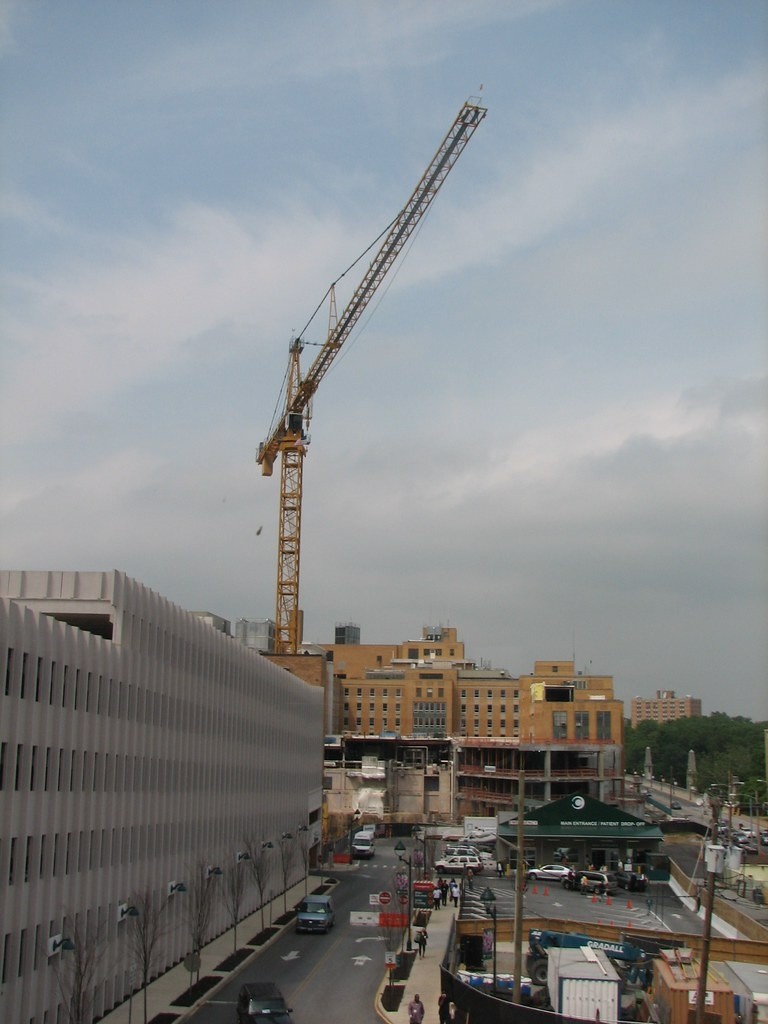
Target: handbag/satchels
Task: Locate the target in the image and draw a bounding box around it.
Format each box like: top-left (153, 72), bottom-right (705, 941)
top-left (414, 933), bottom-right (421, 943)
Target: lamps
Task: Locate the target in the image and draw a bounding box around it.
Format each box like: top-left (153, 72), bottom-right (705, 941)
top-left (206, 865), bottom-right (223, 879)
top-left (47, 934), bottom-right (75, 957)
top-left (237, 852), bottom-right (251, 863)
top-left (118, 903), bottom-right (139, 923)
top-left (168, 881), bottom-right (186, 896)
top-left (282, 833), bottom-right (293, 840)
top-left (298, 824), bottom-right (309, 833)
top-left (261, 840), bottom-right (275, 850)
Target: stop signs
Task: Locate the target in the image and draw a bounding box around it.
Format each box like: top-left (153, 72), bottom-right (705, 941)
top-left (378, 891), bottom-right (391, 904)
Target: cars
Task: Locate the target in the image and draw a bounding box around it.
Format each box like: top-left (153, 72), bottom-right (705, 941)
top-left (527, 865), bottom-right (648, 896)
top-left (718, 824), bottom-right (768, 853)
top-left (671, 801), bottom-right (682, 810)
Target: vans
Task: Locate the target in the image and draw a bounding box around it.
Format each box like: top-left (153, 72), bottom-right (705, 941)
top-left (352, 831), bottom-right (376, 860)
top-left (296, 895), bottom-right (336, 933)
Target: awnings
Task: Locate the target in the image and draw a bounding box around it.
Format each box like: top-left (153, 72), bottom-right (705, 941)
top-left (497, 825), bottom-right (664, 838)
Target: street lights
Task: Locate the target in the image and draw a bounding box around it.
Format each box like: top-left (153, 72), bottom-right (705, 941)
top-left (481, 887), bottom-right (498, 996)
top-left (394, 840), bottom-right (413, 952)
top-left (725, 793), bottom-right (754, 852)
top-left (411, 825), bottom-right (427, 879)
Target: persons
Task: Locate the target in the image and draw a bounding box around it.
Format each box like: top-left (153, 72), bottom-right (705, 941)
top-left (624, 859), bottom-right (632, 872)
top-left (496, 860), bottom-right (504, 878)
top-left (432, 877), bottom-right (460, 910)
top-left (588, 862), bottom-right (608, 872)
top-left (408, 994), bottom-right (425, 1024)
top-left (416, 928), bottom-right (428, 960)
top-left (618, 859), bottom-right (622, 872)
top-left (581, 874), bottom-right (588, 895)
top-left (568, 865), bottom-right (576, 893)
top-left (438, 994), bottom-right (457, 1024)
top-left (467, 869), bottom-right (473, 889)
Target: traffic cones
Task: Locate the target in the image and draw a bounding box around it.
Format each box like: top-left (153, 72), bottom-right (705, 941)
top-left (628, 899), bottom-right (632, 908)
top-left (606, 896), bottom-right (612, 905)
top-left (544, 885), bottom-right (549, 897)
top-left (592, 896), bottom-right (597, 903)
top-left (610, 920), bottom-right (615, 925)
top-left (532, 885), bottom-right (537, 895)
top-left (627, 920), bottom-right (633, 928)
top-left (597, 919), bottom-right (601, 924)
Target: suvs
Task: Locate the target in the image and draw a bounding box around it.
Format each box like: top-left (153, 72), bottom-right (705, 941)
top-left (433, 844), bottom-right (484, 874)
top-left (236, 981), bottom-right (295, 1024)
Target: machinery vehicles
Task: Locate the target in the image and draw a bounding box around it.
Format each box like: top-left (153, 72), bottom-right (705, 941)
top-left (525, 927), bottom-right (683, 985)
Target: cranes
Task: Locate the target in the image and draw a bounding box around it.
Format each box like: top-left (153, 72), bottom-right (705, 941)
top-left (255, 89), bottom-right (491, 654)
